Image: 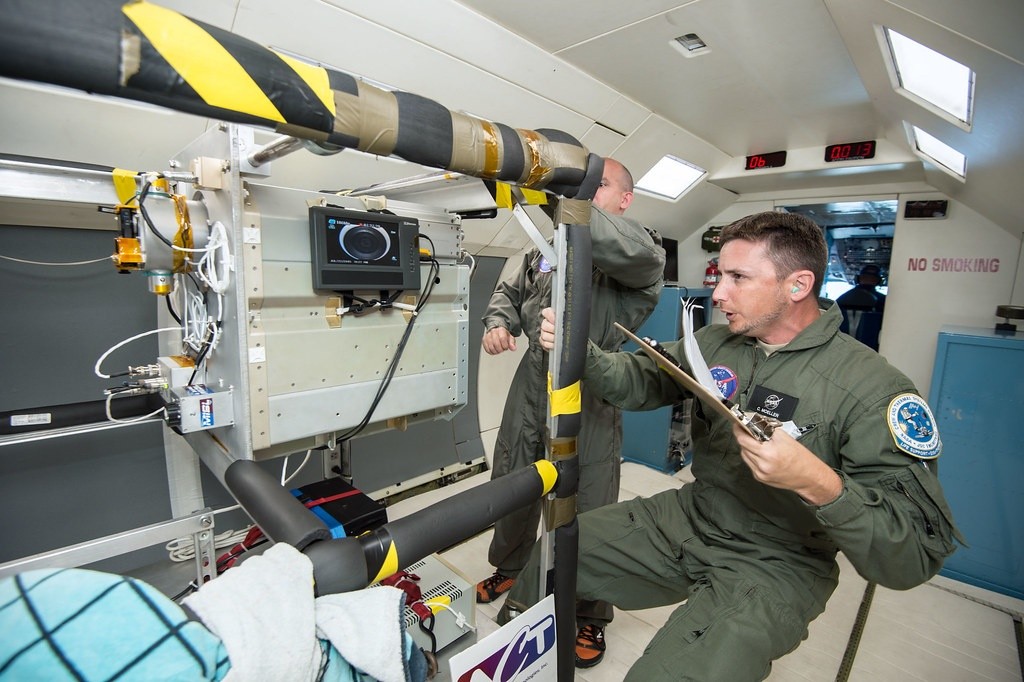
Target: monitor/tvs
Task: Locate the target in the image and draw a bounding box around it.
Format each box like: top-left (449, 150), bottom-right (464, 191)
top-left (308, 206), bottom-right (421, 290)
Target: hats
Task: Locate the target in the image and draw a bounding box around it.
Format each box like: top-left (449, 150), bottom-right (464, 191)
top-left (859, 265), bottom-right (880, 280)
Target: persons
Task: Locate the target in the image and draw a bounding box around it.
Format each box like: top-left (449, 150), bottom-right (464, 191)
top-left (832, 261), bottom-right (890, 358)
top-left (472, 155), bottom-right (667, 670)
top-left (534, 211), bottom-right (970, 682)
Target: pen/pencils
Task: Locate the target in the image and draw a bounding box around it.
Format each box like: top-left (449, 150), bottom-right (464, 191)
top-left (649, 339), bottom-right (685, 370)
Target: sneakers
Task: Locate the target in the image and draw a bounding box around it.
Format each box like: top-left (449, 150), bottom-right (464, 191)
top-left (574, 622), bottom-right (606, 669)
top-left (477, 573), bottom-right (515, 601)
